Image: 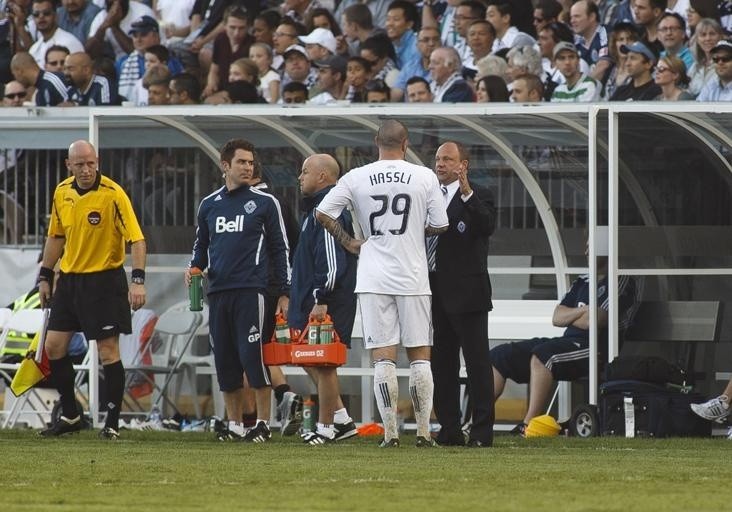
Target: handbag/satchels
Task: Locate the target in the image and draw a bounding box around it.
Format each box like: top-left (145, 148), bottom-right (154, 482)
top-left (598, 354), bottom-right (712, 437)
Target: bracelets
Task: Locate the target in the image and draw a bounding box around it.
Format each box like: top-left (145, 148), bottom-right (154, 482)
top-left (38, 278), bottom-right (49, 282)
top-left (131, 269), bottom-right (145, 279)
top-left (38, 267), bottom-right (51, 277)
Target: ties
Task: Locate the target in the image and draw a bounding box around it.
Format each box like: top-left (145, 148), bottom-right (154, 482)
top-left (426, 186), bottom-right (447, 271)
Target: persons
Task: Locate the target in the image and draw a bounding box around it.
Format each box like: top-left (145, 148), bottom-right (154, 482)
top-left (284, 152), bottom-right (359, 447)
top-left (314, 118), bottom-right (450, 449)
top-left (241, 159), bottom-right (303, 437)
top-left (185, 138), bottom-right (293, 442)
top-left (689, 372), bottom-right (732, 439)
top-left (0, 252), bottom-right (44, 430)
top-left (422, 139), bottom-right (499, 448)
top-left (34, 138), bottom-right (148, 443)
top-left (459, 236), bottom-right (641, 437)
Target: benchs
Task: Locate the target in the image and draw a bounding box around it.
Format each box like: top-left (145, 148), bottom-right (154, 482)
top-left (195, 296), bottom-right (723, 442)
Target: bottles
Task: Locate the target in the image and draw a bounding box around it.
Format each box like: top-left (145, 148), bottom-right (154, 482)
top-left (274, 312), bottom-right (291, 343)
top-left (188, 266), bottom-right (204, 312)
top-left (319, 314), bottom-right (335, 345)
top-left (302, 399), bottom-right (318, 430)
top-left (308, 316), bottom-right (320, 345)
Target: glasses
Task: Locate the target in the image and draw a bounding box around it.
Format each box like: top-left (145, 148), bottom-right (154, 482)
top-left (5, 91), bottom-right (27, 98)
top-left (49, 60), bottom-right (65, 64)
top-left (32, 10), bottom-right (51, 17)
top-left (653, 66), bottom-right (672, 73)
top-left (712, 56), bottom-right (732, 63)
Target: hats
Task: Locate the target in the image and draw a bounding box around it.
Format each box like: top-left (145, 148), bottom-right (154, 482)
top-left (709, 39), bottom-right (732, 53)
top-left (620, 40), bottom-right (657, 64)
top-left (128, 15), bottom-right (159, 35)
top-left (283, 27), bottom-right (338, 63)
top-left (552, 41), bottom-right (577, 60)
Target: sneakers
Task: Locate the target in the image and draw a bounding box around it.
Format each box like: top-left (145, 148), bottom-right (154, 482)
top-left (300, 417), bottom-right (358, 445)
top-left (688, 395), bottom-right (732, 424)
top-left (118, 419), bottom-right (228, 433)
top-left (215, 421), bottom-right (272, 443)
top-left (276, 391), bottom-right (303, 438)
top-left (97, 427), bottom-right (120, 441)
top-left (375, 435), bottom-right (493, 447)
top-left (41, 415), bottom-right (81, 436)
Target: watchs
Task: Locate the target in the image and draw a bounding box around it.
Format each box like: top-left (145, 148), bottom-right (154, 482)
top-left (132, 278), bottom-right (144, 284)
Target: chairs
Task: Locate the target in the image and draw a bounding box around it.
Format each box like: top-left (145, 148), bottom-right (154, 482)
top-left (1, 297), bottom-right (210, 430)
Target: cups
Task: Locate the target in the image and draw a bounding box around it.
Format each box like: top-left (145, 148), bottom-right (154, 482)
top-left (120, 101), bottom-right (136, 107)
